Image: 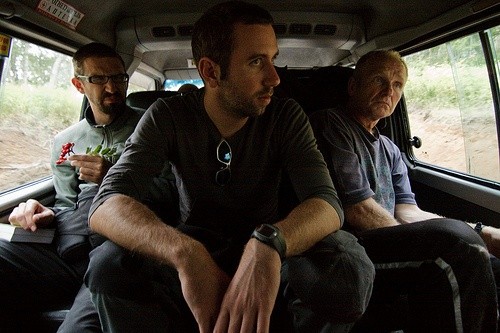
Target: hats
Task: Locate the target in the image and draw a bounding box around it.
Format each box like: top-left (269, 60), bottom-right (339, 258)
top-left (290, 230), bottom-right (375, 324)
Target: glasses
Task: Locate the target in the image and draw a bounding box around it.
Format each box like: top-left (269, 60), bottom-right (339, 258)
top-left (77, 72), bottom-right (129, 84)
top-left (216, 138), bottom-right (232, 192)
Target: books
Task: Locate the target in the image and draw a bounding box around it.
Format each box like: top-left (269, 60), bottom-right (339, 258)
top-left (0, 223), bottom-right (56, 244)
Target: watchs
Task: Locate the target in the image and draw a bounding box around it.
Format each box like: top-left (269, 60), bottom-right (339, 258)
top-left (250, 223), bottom-right (287, 264)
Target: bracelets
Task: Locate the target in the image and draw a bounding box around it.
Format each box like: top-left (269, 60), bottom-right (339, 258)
top-left (475, 222), bottom-right (486, 233)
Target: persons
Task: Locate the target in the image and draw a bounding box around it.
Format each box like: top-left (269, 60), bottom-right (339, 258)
top-left (84, 0), bottom-right (376, 333)
top-left (178, 84), bottom-right (199, 92)
top-left (309, 48), bottom-right (500, 333)
top-left (0, 42), bottom-right (146, 333)
